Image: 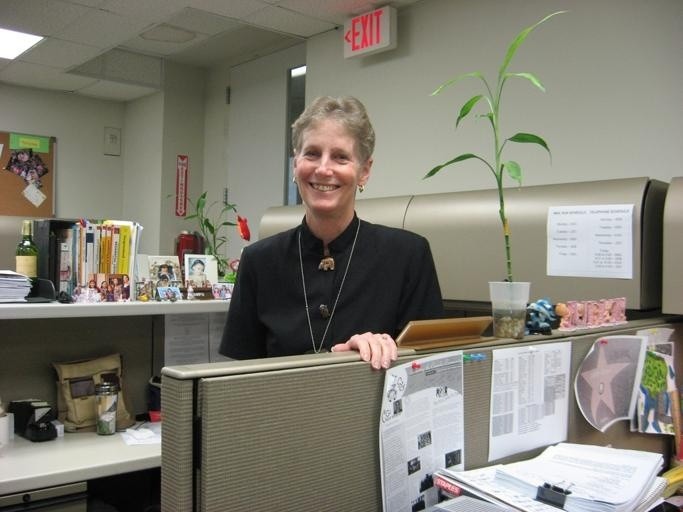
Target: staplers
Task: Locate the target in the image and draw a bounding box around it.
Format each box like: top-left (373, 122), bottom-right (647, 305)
top-left (59, 291), bottom-right (71, 304)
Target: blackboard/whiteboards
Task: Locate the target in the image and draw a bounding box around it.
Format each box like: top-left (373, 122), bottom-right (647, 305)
top-left (0, 130), bottom-right (56, 219)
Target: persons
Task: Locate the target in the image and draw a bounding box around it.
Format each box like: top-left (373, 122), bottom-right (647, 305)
top-left (88, 273), bottom-right (129, 302)
top-left (224, 289), bottom-right (231, 299)
top-left (213, 286), bottom-right (219, 299)
top-left (216, 94), bottom-right (442, 370)
top-left (219, 285), bottom-right (227, 298)
top-left (188, 260), bottom-right (205, 275)
top-left (159, 265), bottom-right (174, 280)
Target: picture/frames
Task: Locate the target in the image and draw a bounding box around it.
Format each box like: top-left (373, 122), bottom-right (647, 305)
top-left (182, 252), bottom-right (218, 290)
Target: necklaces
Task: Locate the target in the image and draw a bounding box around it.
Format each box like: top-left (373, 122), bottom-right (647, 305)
top-left (297, 217), bottom-right (360, 354)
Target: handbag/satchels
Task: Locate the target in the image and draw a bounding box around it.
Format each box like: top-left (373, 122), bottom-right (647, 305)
top-left (48, 352), bottom-right (137, 433)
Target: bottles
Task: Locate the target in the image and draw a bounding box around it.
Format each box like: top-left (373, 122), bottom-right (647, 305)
top-left (93, 379), bottom-right (119, 436)
top-left (15, 219), bottom-right (40, 298)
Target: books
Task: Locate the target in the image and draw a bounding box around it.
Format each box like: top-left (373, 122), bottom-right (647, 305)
top-left (46, 216), bottom-right (144, 299)
top-left (0, 268), bottom-right (34, 302)
top-left (429, 441), bottom-right (670, 512)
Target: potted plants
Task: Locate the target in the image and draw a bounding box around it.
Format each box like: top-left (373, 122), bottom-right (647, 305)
top-left (421, 8), bottom-right (568, 340)
